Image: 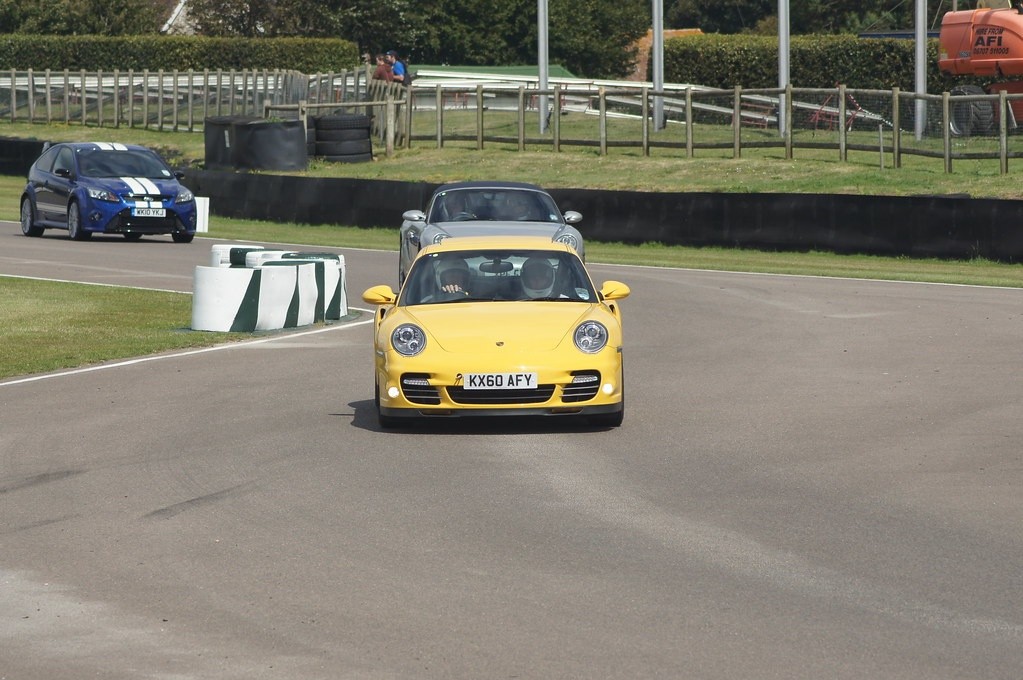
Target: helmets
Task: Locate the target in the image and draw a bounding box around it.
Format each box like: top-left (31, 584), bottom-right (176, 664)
top-left (435, 259), bottom-right (470, 291)
top-left (519, 257), bottom-right (556, 299)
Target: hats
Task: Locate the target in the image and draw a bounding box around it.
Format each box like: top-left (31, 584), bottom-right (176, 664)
top-left (385, 49), bottom-right (398, 57)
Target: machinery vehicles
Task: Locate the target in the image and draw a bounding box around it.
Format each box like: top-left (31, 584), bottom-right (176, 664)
top-left (937, 0), bottom-right (1023, 137)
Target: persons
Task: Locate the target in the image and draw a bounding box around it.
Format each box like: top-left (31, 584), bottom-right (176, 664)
top-left (421, 255), bottom-right (473, 304)
top-left (510, 256), bottom-right (561, 302)
top-left (385, 50), bottom-right (406, 86)
top-left (372, 54), bottom-right (394, 83)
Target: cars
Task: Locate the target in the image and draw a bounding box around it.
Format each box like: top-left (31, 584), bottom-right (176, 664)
top-left (17, 139), bottom-right (202, 243)
top-left (361, 235), bottom-right (630, 425)
top-left (396, 178), bottom-right (585, 290)
